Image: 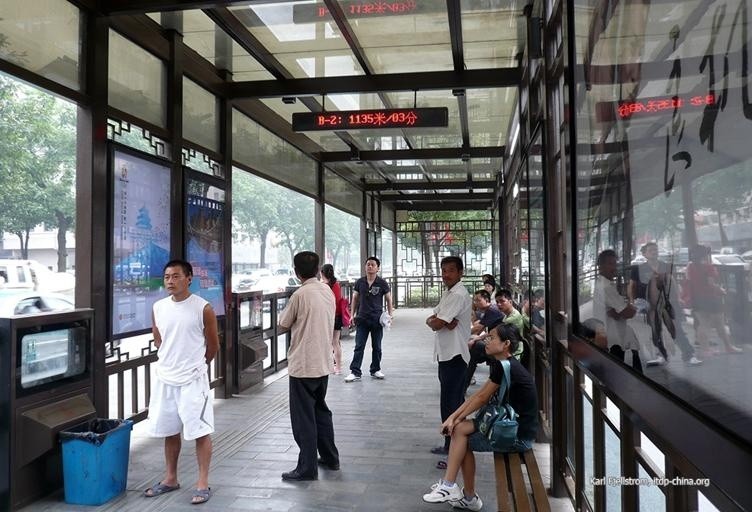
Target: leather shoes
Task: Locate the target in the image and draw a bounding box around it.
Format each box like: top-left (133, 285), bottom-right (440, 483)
top-left (282, 469), bottom-right (318, 481)
top-left (318, 459), bottom-right (340, 470)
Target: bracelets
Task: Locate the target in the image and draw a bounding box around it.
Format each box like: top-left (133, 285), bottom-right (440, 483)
top-left (390, 317), bottom-right (394, 320)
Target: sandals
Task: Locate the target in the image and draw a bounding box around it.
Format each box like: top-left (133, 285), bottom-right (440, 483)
top-left (435, 457), bottom-right (448, 469)
top-left (431, 446), bottom-right (449, 455)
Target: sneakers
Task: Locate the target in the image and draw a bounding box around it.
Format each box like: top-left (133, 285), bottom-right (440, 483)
top-left (334, 367), bottom-right (341, 375)
top-left (447, 486), bottom-right (483, 511)
top-left (371, 371), bottom-right (384, 380)
top-left (727, 347), bottom-right (743, 353)
top-left (423, 478), bottom-right (464, 503)
top-left (344, 374), bottom-right (360, 382)
top-left (687, 357), bottom-right (702, 366)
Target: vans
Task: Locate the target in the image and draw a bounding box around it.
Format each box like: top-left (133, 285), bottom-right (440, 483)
top-left (345, 264), bottom-right (362, 285)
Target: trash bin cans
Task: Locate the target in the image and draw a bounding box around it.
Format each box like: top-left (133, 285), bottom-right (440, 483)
top-left (58, 418), bottom-right (134, 506)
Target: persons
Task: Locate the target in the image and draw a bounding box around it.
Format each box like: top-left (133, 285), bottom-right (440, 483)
top-left (144, 260), bottom-right (221, 504)
top-left (685, 244), bottom-right (743, 357)
top-left (532, 288), bottom-right (545, 330)
top-left (278, 250), bottom-right (340, 480)
top-left (319, 264), bottom-right (344, 375)
top-left (468, 273), bottom-right (523, 386)
top-left (421, 322), bottom-right (539, 512)
top-left (629, 240), bottom-right (704, 365)
top-left (426, 257), bottom-right (472, 469)
top-left (343, 257), bottom-right (394, 383)
top-left (592, 250), bottom-right (642, 376)
top-left (583, 317), bottom-right (608, 351)
top-left (520, 288), bottom-right (535, 323)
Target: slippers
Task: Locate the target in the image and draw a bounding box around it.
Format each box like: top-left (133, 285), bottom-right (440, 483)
top-left (143, 481), bottom-right (180, 497)
top-left (190, 487), bottom-right (210, 504)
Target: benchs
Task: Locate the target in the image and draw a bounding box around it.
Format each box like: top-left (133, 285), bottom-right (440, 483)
top-left (492, 446), bottom-right (552, 512)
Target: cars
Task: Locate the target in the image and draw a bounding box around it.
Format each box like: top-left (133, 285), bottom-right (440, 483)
top-left (0, 260), bottom-right (53, 286)
top-left (0, 286), bottom-right (74, 315)
top-left (234, 267), bottom-right (300, 313)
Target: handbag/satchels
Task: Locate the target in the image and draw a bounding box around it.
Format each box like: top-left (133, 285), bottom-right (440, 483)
top-left (340, 296), bottom-right (351, 326)
top-left (475, 396), bottom-right (520, 453)
top-left (680, 279), bottom-right (693, 309)
